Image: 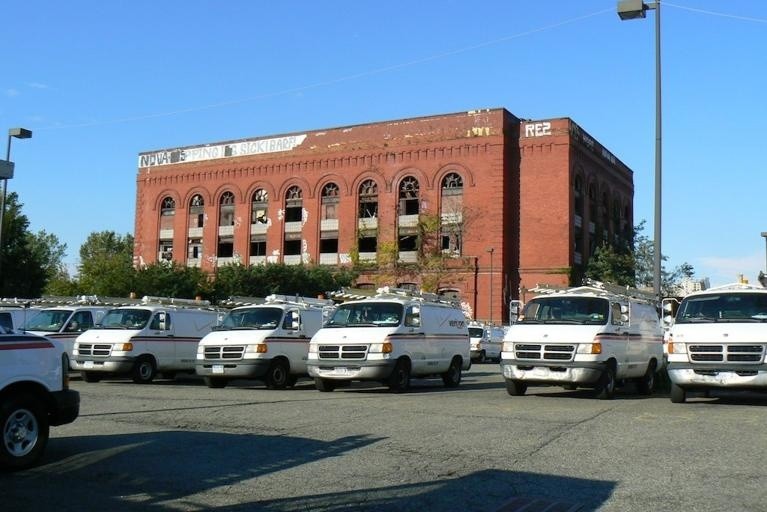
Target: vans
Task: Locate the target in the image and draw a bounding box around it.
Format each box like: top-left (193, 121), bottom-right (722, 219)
top-left (307, 299), bottom-right (472, 388)
top-left (467, 325), bottom-right (509, 362)
top-left (661, 283), bottom-right (767, 404)
top-left (195, 303), bottom-right (348, 390)
top-left (501, 292), bottom-right (663, 398)
top-left (20, 307), bottom-right (112, 370)
top-left (70, 305), bottom-right (227, 384)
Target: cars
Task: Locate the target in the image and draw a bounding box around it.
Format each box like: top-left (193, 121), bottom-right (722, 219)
top-left (1, 328), bottom-right (79, 461)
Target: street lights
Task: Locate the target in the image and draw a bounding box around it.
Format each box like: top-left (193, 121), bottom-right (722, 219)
top-left (1, 128), bottom-right (31, 237)
top-left (486, 247), bottom-right (494, 324)
top-left (618, 0), bottom-right (661, 301)
